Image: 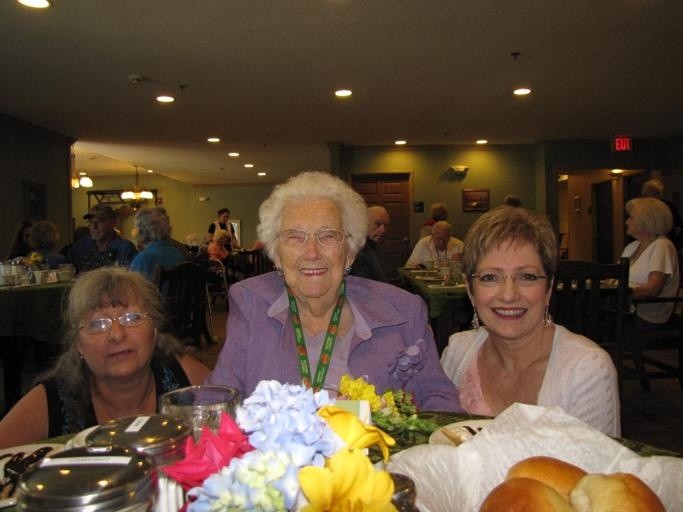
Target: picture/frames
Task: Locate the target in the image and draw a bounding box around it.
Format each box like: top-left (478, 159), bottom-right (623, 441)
top-left (461, 188), bottom-right (490, 213)
top-left (228, 218), bottom-right (241, 252)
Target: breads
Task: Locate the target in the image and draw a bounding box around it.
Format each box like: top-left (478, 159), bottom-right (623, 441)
top-left (479, 478), bottom-right (575, 512)
top-left (570, 473), bottom-right (666, 512)
top-left (506, 456), bottom-right (588, 502)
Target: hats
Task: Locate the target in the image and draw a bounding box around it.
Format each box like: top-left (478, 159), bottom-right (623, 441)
top-left (83, 204), bottom-right (117, 221)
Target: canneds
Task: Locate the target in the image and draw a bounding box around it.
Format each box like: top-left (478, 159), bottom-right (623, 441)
top-left (10, 261), bottom-right (26, 276)
top-left (0, 260), bottom-right (11, 274)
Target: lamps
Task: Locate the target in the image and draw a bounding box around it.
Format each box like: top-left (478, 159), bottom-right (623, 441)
top-left (119, 167), bottom-right (153, 202)
top-left (70, 152), bottom-right (93, 189)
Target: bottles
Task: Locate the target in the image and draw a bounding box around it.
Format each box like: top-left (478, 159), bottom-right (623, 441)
top-left (10, 259), bottom-right (25, 285)
top-left (0, 260), bottom-right (10, 286)
top-left (19, 443), bottom-right (161, 512)
top-left (390, 471), bottom-right (420, 511)
top-left (159, 383), bottom-right (241, 436)
top-left (84, 414), bottom-right (195, 512)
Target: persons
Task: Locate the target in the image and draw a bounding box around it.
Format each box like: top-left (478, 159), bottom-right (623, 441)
top-left (65, 204), bottom-right (137, 275)
top-left (403, 221), bottom-right (465, 268)
top-left (59, 227), bottom-right (89, 256)
top-left (420, 203), bottom-right (447, 238)
top-left (126, 214), bottom-right (198, 339)
top-left (347, 206), bottom-right (412, 293)
top-left (0, 267), bottom-right (212, 449)
top-left (622, 178), bottom-right (678, 254)
top-left (25, 221), bottom-right (64, 269)
top-left (204, 208), bottom-right (237, 279)
top-left (138, 205), bottom-right (194, 260)
top-left (5, 219), bottom-right (35, 263)
top-left (190, 170), bottom-right (467, 424)
top-left (577, 197), bottom-right (679, 368)
top-left (206, 230), bottom-right (239, 284)
top-left (438, 205), bottom-right (621, 440)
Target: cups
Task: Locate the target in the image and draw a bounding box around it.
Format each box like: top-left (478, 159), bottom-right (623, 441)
top-left (33, 270), bottom-right (48, 285)
top-left (56, 270), bottom-right (73, 283)
top-left (423, 258), bottom-right (434, 270)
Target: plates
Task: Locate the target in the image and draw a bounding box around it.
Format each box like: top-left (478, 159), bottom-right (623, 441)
top-left (427, 419), bottom-right (493, 448)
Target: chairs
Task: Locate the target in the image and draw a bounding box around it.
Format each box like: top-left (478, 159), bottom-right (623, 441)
top-left (152, 263), bottom-right (204, 351)
top-left (625, 285), bottom-right (682, 392)
top-left (246, 247), bottom-right (273, 276)
top-left (552, 262), bottom-right (630, 392)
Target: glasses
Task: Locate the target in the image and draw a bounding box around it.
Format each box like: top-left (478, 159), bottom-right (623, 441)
top-left (469, 270), bottom-right (549, 286)
top-left (272, 227), bottom-right (352, 249)
top-left (73, 311), bottom-right (162, 337)
